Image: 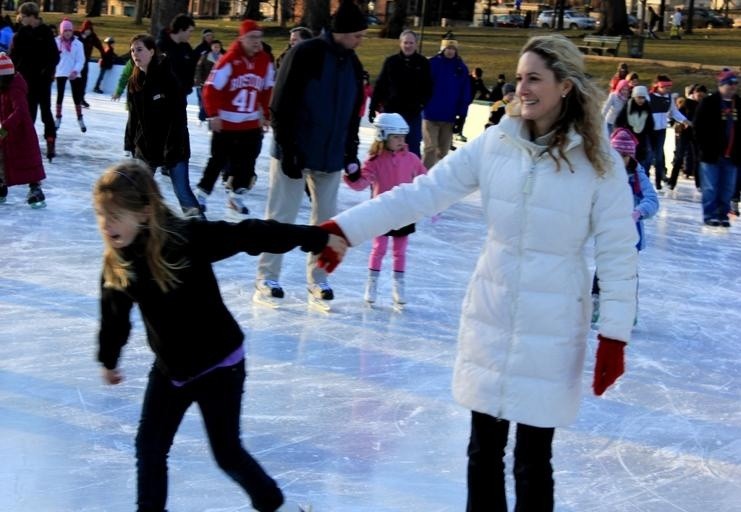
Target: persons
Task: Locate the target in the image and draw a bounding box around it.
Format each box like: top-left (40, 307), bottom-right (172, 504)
top-left (112, 13), bottom-right (311, 222)
top-left (91, 155), bottom-right (349, 512)
top-left (253, 0), bottom-right (371, 303)
top-left (367, 30), bottom-right (516, 169)
top-left (341, 109), bottom-right (444, 307)
top-left (524, 4), bottom-right (590, 28)
top-left (602, 61), bottom-right (741, 228)
top-left (648, 7), bottom-right (682, 40)
top-left (309, 32), bottom-right (644, 511)
top-left (1, 1), bottom-right (120, 210)
top-left (590, 127), bottom-right (661, 332)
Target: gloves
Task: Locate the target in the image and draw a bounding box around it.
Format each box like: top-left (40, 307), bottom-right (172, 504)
top-left (277, 146), bottom-right (304, 179)
top-left (301, 222), bottom-right (349, 274)
top-left (344, 156), bottom-right (362, 181)
top-left (592, 334), bottom-right (626, 395)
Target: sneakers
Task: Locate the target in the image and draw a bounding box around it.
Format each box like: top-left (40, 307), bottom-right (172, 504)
top-left (27, 189), bottom-right (45, 204)
top-left (393, 280), bottom-right (406, 304)
top-left (364, 277), bottom-right (379, 301)
top-left (194, 189), bottom-right (207, 212)
top-left (46, 137), bottom-right (55, 156)
top-left (76, 113), bottom-right (86, 132)
top-left (229, 192), bottom-right (250, 214)
top-left (257, 279), bottom-right (285, 300)
top-left (707, 218), bottom-right (730, 227)
top-left (55, 115), bottom-right (61, 127)
top-left (307, 279), bottom-right (333, 301)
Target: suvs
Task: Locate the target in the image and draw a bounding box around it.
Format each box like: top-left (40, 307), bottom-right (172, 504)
top-left (536, 10), bottom-right (595, 30)
top-left (680, 7), bottom-right (734, 30)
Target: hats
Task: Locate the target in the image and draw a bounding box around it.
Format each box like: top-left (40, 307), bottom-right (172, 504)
top-left (330, 2), bottom-right (369, 34)
top-left (716, 67), bottom-right (738, 86)
top-left (202, 29), bottom-right (214, 38)
top-left (440, 32), bottom-right (458, 51)
top-left (60, 18), bottom-right (75, 38)
top-left (610, 128), bottom-right (637, 157)
top-left (0, 52), bottom-right (15, 74)
top-left (240, 20), bottom-right (262, 38)
top-left (617, 63), bottom-right (673, 101)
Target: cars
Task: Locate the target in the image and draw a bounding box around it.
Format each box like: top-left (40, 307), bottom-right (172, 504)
top-left (596, 13), bottom-right (649, 29)
top-left (262, 17), bottom-right (276, 22)
top-left (369, 13), bottom-right (385, 24)
top-left (495, 14), bottom-right (538, 28)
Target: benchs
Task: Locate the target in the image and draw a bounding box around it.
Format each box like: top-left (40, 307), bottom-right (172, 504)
top-left (576, 33), bottom-right (622, 59)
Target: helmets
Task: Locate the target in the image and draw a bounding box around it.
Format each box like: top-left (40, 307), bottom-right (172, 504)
top-left (104, 36), bottom-right (114, 43)
top-left (372, 113), bottom-right (410, 142)
